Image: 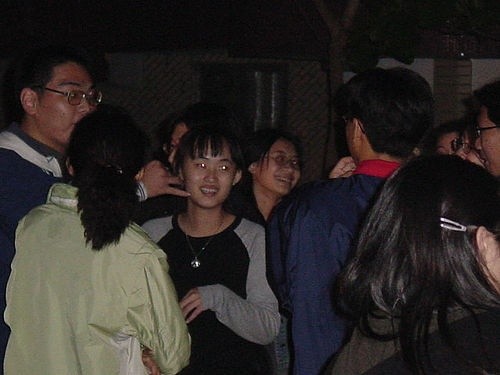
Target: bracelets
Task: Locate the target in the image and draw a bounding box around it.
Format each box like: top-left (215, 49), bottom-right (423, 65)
top-left (138, 182), bottom-right (147, 200)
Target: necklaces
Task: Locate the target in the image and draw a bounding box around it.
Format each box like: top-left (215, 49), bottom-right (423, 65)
top-left (183, 215), bottom-right (224, 268)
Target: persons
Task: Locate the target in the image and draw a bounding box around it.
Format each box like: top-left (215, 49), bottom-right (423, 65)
top-left (140, 128), bottom-right (281, 375)
top-left (137, 103), bottom-right (229, 227)
top-left (0, 55), bottom-right (191, 375)
top-left (4, 107), bottom-right (192, 374)
top-left (425, 79), bottom-right (500, 177)
top-left (326, 151), bottom-right (500, 375)
top-left (226, 131), bottom-right (300, 375)
top-left (271, 65), bottom-right (432, 375)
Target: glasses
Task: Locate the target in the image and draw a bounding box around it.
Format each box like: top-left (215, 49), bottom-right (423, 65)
top-left (261, 154), bottom-right (303, 170)
top-left (38, 87), bottom-right (103, 107)
top-left (450, 137), bottom-right (472, 154)
top-left (470, 125), bottom-right (499, 138)
